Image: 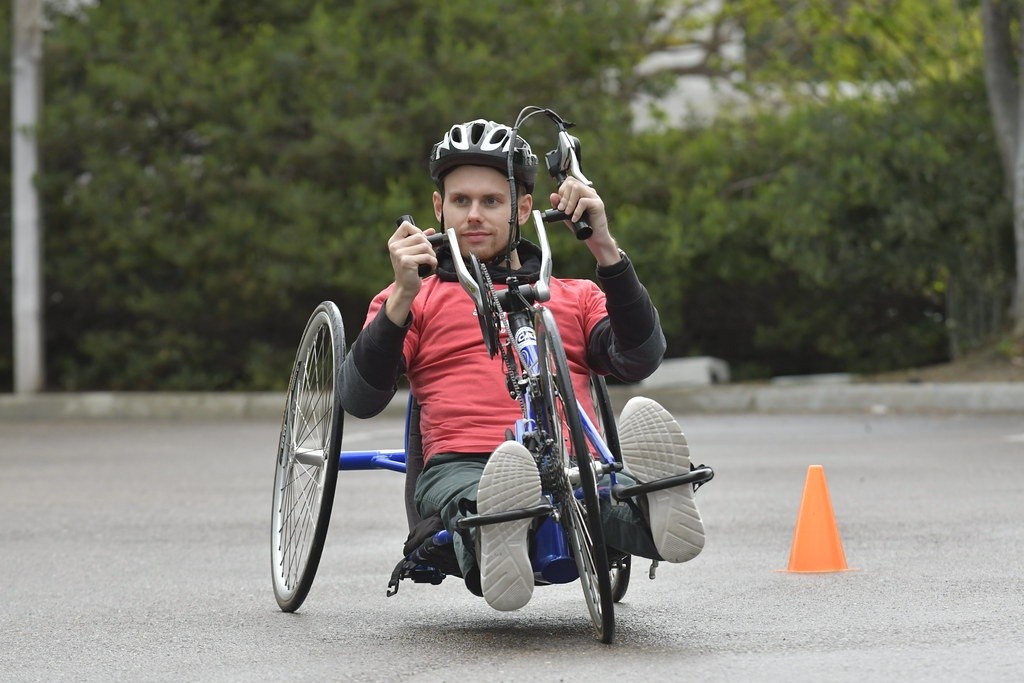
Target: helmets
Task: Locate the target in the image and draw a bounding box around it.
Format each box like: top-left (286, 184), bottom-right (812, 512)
top-left (429, 118), bottom-right (539, 194)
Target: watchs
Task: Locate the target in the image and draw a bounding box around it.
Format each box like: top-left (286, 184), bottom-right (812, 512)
top-left (596, 247), bottom-right (629, 277)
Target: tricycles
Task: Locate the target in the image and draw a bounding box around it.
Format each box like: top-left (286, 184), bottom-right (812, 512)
top-left (271, 106), bottom-right (715, 644)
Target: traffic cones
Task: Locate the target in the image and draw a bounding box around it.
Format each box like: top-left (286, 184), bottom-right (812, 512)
top-left (777, 464), bottom-right (861, 574)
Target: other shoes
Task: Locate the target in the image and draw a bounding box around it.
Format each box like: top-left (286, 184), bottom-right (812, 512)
top-left (474, 440), bottom-right (542, 612)
top-left (617, 396), bottom-right (705, 563)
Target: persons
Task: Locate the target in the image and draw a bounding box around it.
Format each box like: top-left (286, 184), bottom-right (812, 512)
top-left (335, 119), bottom-right (705, 611)
